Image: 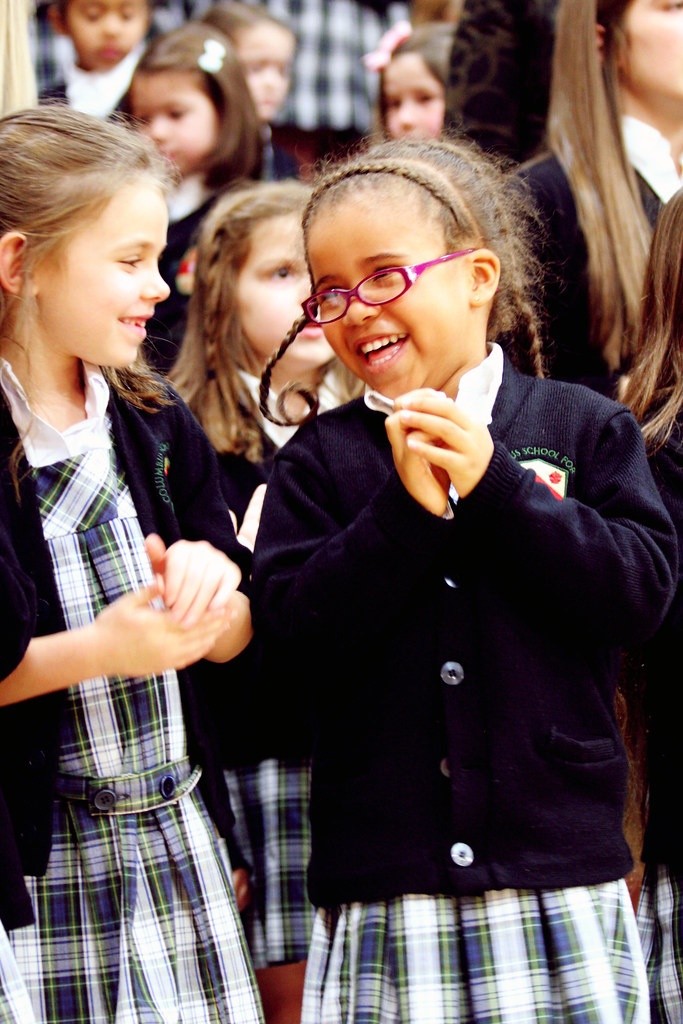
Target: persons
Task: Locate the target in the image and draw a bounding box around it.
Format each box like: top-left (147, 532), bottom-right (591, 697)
top-left (607, 187), bottom-right (683, 1023)
top-left (487, 0), bottom-right (682, 403)
top-left (122, 21), bottom-right (264, 375)
top-left (0, 97), bottom-right (267, 1023)
top-left (164, 176), bottom-right (367, 1024)
top-left (198, 0), bottom-right (304, 182)
top-left (367, 20), bottom-right (459, 139)
top-left (143, 135), bottom-right (683, 1024)
top-left (30, 0), bottom-right (167, 122)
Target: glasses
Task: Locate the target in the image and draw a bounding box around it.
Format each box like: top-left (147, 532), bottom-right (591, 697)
top-left (302, 246), bottom-right (476, 325)
